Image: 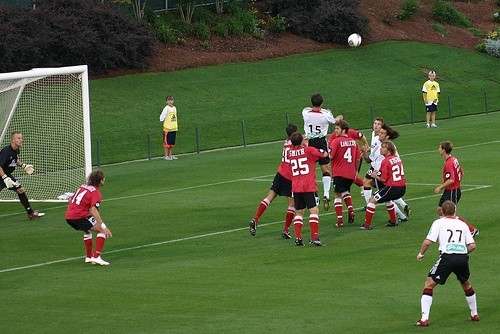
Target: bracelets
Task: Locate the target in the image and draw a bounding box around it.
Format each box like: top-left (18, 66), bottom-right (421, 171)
top-left (100, 223), bottom-right (107, 230)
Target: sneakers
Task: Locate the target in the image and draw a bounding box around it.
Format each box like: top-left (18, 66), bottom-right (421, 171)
top-left (397, 217), bottom-right (408, 222)
top-left (335, 222), bottom-right (344, 227)
top-left (404, 205), bottom-right (412, 217)
top-left (281, 232), bottom-right (291, 239)
top-left (348, 213), bottom-right (355, 223)
top-left (414, 319), bottom-right (429, 326)
top-left (322, 196), bottom-right (330, 211)
top-left (295, 238), bottom-right (304, 246)
top-left (249, 219), bottom-right (257, 236)
top-left (467, 314), bottom-right (480, 321)
top-left (309, 239), bottom-right (327, 246)
top-left (314, 194), bottom-right (321, 207)
top-left (362, 206), bottom-right (366, 211)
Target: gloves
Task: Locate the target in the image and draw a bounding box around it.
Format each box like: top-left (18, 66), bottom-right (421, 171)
top-left (2, 174), bottom-right (17, 188)
top-left (20, 163), bottom-right (34, 175)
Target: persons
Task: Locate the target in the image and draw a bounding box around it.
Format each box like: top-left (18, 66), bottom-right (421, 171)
top-left (285, 133), bottom-right (327, 246)
top-left (250, 124), bottom-right (298, 240)
top-left (435, 142), bottom-right (478, 240)
top-left (421, 71), bottom-right (441, 129)
top-left (417, 200), bottom-right (478, 327)
top-left (0, 131), bottom-right (45, 220)
top-left (302, 93), bottom-right (411, 230)
top-left (160, 95), bottom-right (179, 161)
top-left (65, 170), bottom-right (113, 265)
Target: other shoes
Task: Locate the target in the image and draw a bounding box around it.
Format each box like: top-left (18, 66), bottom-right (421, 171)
top-left (164, 155), bottom-right (178, 160)
top-left (28, 211), bottom-right (45, 220)
top-left (360, 224), bottom-right (372, 230)
top-left (384, 220), bottom-right (399, 227)
top-left (85, 255), bottom-right (109, 266)
top-left (426, 124), bottom-right (437, 128)
top-left (470, 228), bottom-right (479, 238)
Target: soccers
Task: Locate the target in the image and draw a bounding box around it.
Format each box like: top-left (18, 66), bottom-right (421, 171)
top-left (347, 33), bottom-right (362, 48)
